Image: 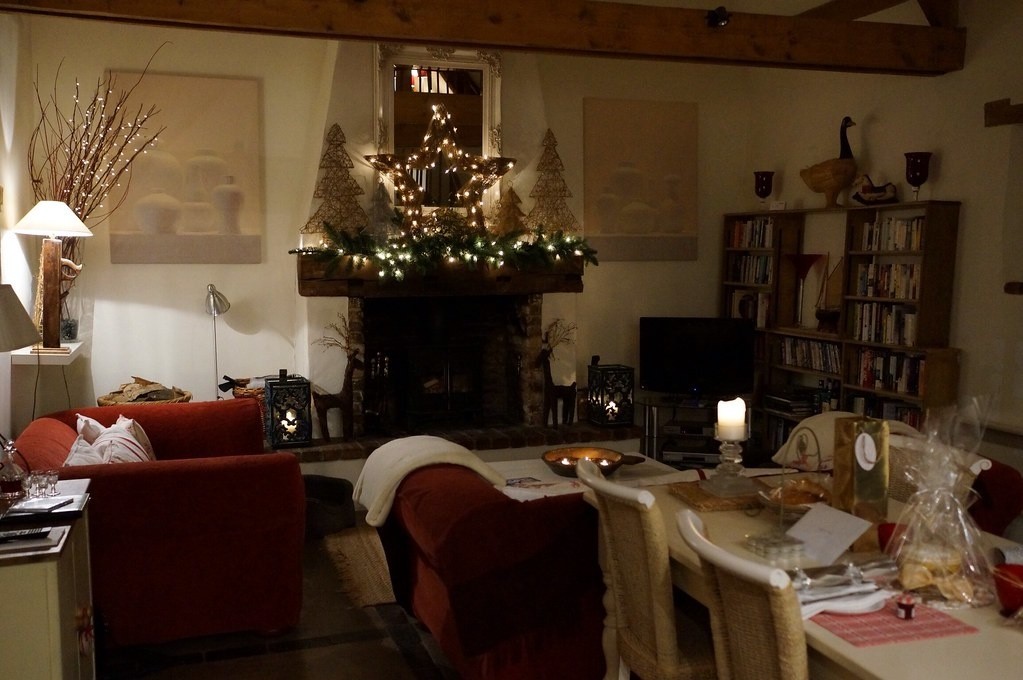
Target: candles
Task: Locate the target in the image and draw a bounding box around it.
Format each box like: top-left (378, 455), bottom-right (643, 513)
top-left (716, 396), bottom-right (746, 441)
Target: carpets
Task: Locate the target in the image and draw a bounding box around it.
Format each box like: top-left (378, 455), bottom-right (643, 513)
top-left (323, 521), bottom-right (396, 607)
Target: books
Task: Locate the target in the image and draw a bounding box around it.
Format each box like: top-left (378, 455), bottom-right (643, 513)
top-left (763, 392), bottom-right (815, 416)
top-left (781, 336), bottom-right (844, 376)
top-left (715, 216), bottom-right (772, 328)
top-left (9, 496), bottom-right (74, 512)
top-left (845, 215), bottom-right (924, 428)
top-left (0, 527), bottom-right (67, 553)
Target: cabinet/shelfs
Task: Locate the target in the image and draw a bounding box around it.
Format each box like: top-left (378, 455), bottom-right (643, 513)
top-left (718, 196), bottom-right (966, 443)
top-left (635, 395), bottom-right (758, 464)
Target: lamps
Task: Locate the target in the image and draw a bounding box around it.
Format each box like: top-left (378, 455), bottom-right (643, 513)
top-left (11, 201), bottom-right (94, 352)
top-left (264, 369), bottom-right (314, 446)
top-left (584, 354), bottom-right (638, 430)
top-left (705, 6), bottom-right (734, 28)
top-left (203, 282), bottom-right (231, 403)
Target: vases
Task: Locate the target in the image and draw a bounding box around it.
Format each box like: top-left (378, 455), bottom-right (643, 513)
top-left (60, 297), bottom-right (82, 343)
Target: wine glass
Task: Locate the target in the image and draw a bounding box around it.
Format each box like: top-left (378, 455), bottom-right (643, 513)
top-left (22, 469), bottom-right (59, 500)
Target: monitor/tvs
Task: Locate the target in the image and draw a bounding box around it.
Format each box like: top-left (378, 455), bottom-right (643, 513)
top-left (639, 316), bottom-right (754, 395)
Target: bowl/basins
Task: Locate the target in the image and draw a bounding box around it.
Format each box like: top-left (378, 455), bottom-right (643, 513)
top-left (994, 563), bottom-right (1023, 614)
top-left (543, 447), bottom-right (626, 477)
top-left (878, 523), bottom-right (913, 556)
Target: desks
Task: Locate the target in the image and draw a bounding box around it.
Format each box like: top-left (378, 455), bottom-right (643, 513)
top-left (485, 450), bottom-right (683, 503)
top-left (589, 466), bottom-right (1022, 680)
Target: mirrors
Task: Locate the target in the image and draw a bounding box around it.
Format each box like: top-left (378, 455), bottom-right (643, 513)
top-left (373, 42), bottom-right (503, 223)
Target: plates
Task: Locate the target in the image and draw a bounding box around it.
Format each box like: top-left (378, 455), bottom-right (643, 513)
top-left (829, 602), bottom-right (884, 614)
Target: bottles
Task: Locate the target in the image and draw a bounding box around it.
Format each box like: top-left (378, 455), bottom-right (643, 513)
top-left (0, 438), bottom-right (25, 499)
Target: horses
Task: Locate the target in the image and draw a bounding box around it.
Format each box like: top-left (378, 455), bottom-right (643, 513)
top-left (852, 173), bottom-right (897, 202)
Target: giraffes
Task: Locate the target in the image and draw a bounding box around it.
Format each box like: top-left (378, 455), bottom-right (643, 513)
top-left (312, 351), bottom-right (366, 443)
top-left (533, 348), bottom-right (577, 426)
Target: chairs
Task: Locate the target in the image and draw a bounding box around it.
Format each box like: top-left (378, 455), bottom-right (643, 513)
top-left (673, 506), bottom-right (812, 679)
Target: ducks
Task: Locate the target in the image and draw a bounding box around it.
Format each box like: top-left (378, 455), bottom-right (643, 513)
top-left (800, 116), bottom-right (859, 209)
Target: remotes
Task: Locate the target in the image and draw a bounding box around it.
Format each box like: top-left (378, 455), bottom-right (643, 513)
top-left (0, 527), bottom-right (52, 541)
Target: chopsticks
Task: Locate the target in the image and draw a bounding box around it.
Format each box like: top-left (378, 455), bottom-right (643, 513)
top-left (800, 587), bottom-right (878, 604)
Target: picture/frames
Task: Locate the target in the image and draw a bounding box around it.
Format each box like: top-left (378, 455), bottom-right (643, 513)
top-left (100, 67), bottom-right (265, 265)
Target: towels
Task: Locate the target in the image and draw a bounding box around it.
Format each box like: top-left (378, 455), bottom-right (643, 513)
top-left (351, 435), bottom-right (506, 527)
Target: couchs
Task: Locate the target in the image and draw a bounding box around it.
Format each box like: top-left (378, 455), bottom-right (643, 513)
top-left (351, 433), bottom-right (605, 680)
top-left (19, 397), bottom-right (309, 649)
top-left (945, 452), bottom-right (1023, 538)
top-left (574, 457), bottom-right (717, 680)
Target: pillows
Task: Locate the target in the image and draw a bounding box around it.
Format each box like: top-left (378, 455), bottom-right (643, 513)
top-left (62, 433), bottom-right (103, 465)
top-left (116, 413), bottom-right (155, 462)
top-left (73, 411), bottom-right (108, 444)
top-left (102, 434), bottom-right (152, 462)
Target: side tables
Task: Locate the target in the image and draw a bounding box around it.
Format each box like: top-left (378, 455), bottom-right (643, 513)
top-left (0, 476), bottom-right (109, 680)
